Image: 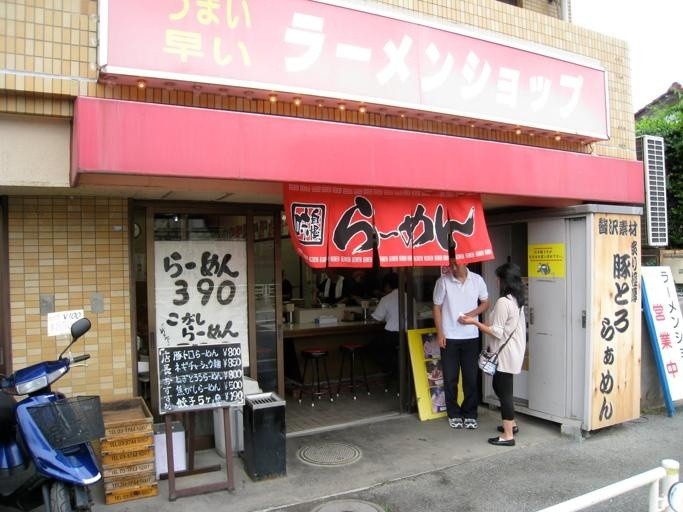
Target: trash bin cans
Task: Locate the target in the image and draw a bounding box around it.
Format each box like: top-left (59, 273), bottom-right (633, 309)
top-left (242, 391), bottom-right (288, 484)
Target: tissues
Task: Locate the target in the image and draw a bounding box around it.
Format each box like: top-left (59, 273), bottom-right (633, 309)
top-left (319, 315), bottom-right (337, 323)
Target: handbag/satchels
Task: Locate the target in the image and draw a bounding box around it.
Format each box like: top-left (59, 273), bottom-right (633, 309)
top-left (477, 349), bottom-right (501, 377)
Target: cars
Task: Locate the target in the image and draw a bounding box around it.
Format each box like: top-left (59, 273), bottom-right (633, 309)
top-left (255, 298), bottom-right (276, 388)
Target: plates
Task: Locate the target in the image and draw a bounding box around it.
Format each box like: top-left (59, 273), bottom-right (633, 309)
top-left (431, 393), bottom-right (447, 406)
top-left (429, 375), bottom-right (443, 381)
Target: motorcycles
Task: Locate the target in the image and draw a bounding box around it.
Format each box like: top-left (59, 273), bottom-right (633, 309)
top-left (1, 316), bottom-right (104, 510)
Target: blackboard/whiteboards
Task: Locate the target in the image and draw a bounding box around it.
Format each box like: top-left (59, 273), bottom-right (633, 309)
top-left (157, 343), bottom-right (246, 415)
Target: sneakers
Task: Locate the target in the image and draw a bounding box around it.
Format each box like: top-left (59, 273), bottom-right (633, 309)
top-left (447, 416), bottom-right (463, 429)
top-left (463, 418), bottom-right (479, 429)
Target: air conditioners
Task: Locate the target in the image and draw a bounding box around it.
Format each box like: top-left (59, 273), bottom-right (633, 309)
top-left (634, 136), bottom-right (670, 248)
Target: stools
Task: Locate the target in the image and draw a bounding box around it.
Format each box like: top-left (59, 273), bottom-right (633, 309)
top-left (255, 346), bottom-right (276, 393)
top-left (297, 347), bottom-right (335, 409)
top-left (334, 341), bottom-right (371, 400)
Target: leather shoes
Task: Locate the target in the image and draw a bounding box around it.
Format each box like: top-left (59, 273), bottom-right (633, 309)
top-left (497, 425), bottom-right (518, 434)
top-left (487, 436), bottom-right (515, 446)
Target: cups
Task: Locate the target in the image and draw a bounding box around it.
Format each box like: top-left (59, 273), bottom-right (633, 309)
top-left (362, 308), bottom-right (370, 320)
top-left (287, 311), bottom-right (296, 324)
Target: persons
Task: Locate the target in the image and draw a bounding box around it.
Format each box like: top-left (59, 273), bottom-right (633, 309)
top-left (371, 273), bottom-right (418, 382)
top-left (310, 267), bottom-right (358, 307)
top-left (432, 252), bottom-right (490, 428)
top-left (457, 261), bottom-right (527, 446)
top-left (282, 270), bottom-right (292, 301)
top-left (255, 284), bottom-right (277, 349)
